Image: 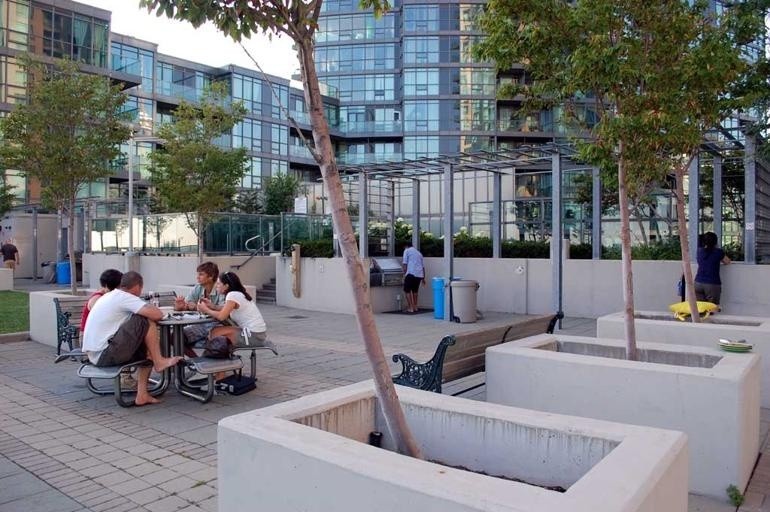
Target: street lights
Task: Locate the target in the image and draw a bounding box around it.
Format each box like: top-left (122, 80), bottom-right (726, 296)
top-left (125, 123), bottom-right (141, 274)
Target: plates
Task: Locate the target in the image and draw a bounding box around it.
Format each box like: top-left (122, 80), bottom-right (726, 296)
top-left (721, 343), bottom-right (753, 353)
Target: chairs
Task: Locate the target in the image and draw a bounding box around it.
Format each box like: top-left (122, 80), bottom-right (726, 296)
top-left (390, 309), bottom-right (565, 397)
top-left (53, 290), bottom-right (178, 363)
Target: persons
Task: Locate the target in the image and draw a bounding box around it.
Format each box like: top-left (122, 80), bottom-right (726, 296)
top-left (0, 238), bottom-right (19, 271)
top-left (83, 272), bottom-right (183, 404)
top-left (399, 241), bottom-right (425, 314)
top-left (176, 262), bottom-right (227, 358)
top-left (693, 232), bottom-right (732, 306)
top-left (78, 269), bottom-right (124, 348)
top-left (197, 272), bottom-right (266, 383)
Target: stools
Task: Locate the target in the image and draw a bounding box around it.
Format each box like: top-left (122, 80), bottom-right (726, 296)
top-left (54, 348), bottom-right (90, 362)
top-left (78, 358), bottom-right (160, 407)
top-left (178, 353), bottom-right (243, 405)
top-left (229, 338), bottom-right (279, 379)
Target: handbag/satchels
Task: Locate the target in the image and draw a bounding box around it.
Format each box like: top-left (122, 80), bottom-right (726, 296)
top-left (669, 301), bottom-right (721, 320)
top-left (203, 336), bottom-right (234, 360)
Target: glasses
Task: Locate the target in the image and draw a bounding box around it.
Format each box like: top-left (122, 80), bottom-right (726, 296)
top-left (224, 271), bottom-right (232, 283)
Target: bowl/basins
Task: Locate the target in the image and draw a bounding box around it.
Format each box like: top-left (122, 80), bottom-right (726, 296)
top-left (157, 306), bottom-right (175, 315)
